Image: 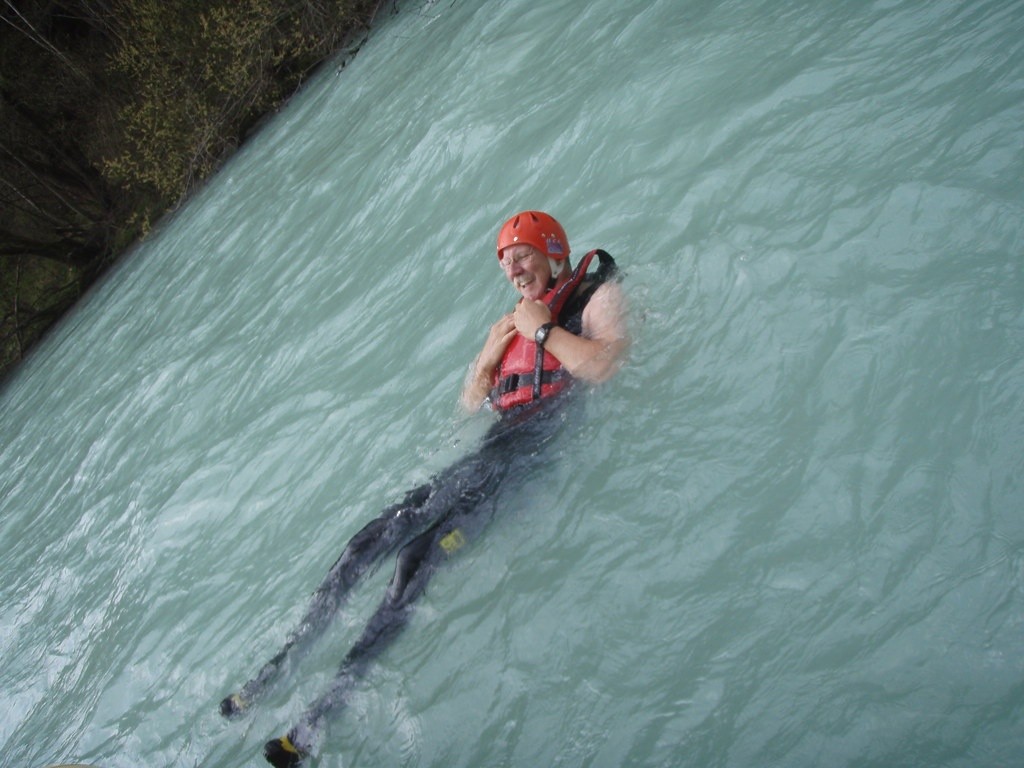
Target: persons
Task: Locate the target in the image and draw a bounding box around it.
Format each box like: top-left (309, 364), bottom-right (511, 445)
top-left (220, 210), bottom-right (627, 768)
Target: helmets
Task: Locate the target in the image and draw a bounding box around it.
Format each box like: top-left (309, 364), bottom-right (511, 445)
top-left (497, 210), bottom-right (571, 260)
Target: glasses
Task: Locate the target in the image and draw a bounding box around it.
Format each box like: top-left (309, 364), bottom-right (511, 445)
top-left (500, 250), bottom-right (537, 270)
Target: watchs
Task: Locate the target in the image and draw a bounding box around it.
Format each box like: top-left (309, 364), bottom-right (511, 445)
top-left (535, 322), bottom-right (559, 350)
